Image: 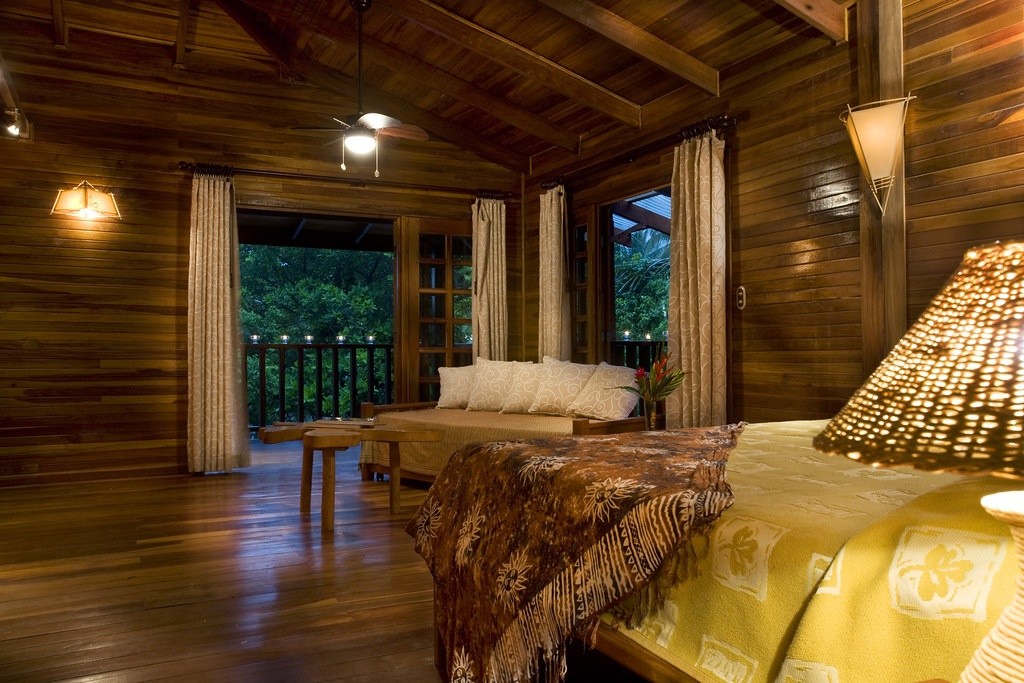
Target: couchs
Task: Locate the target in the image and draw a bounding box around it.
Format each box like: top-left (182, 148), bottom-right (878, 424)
top-left (360, 401), bottom-right (660, 487)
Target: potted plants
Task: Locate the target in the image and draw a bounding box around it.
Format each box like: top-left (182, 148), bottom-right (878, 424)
top-left (604, 353), bottom-right (693, 431)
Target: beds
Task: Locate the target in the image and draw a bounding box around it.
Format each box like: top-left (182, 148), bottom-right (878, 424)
top-left (404, 416), bottom-right (1023, 683)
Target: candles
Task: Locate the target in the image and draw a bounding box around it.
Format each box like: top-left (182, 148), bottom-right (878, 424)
top-left (336, 335), bottom-right (345, 344)
top-left (366, 336), bottom-right (375, 344)
top-left (281, 335), bottom-right (289, 344)
top-left (304, 335), bottom-right (314, 345)
top-left (250, 334), bottom-right (260, 344)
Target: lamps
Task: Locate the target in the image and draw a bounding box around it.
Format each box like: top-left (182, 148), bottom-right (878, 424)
top-left (340, 124), bottom-right (381, 178)
top-left (5, 110), bottom-right (26, 134)
top-left (838, 93), bottom-right (917, 218)
top-left (811, 238), bottom-right (1024, 682)
top-left (50, 179), bottom-right (122, 219)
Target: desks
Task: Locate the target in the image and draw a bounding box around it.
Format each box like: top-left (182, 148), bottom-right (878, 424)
top-left (256, 421), bottom-right (446, 532)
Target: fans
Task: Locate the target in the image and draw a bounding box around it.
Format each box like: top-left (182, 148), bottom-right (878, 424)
top-left (291, 0), bottom-right (428, 146)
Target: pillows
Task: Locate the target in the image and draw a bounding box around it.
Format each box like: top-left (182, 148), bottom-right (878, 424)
top-left (563, 362), bottom-right (641, 421)
top-left (466, 357), bottom-right (532, 412)
top-left (527, 355), bottom-right (598, 416)
top-left (500, 360), bottom-right (543, 414)
top-left (435, 365), bottom-right (476, 410)
